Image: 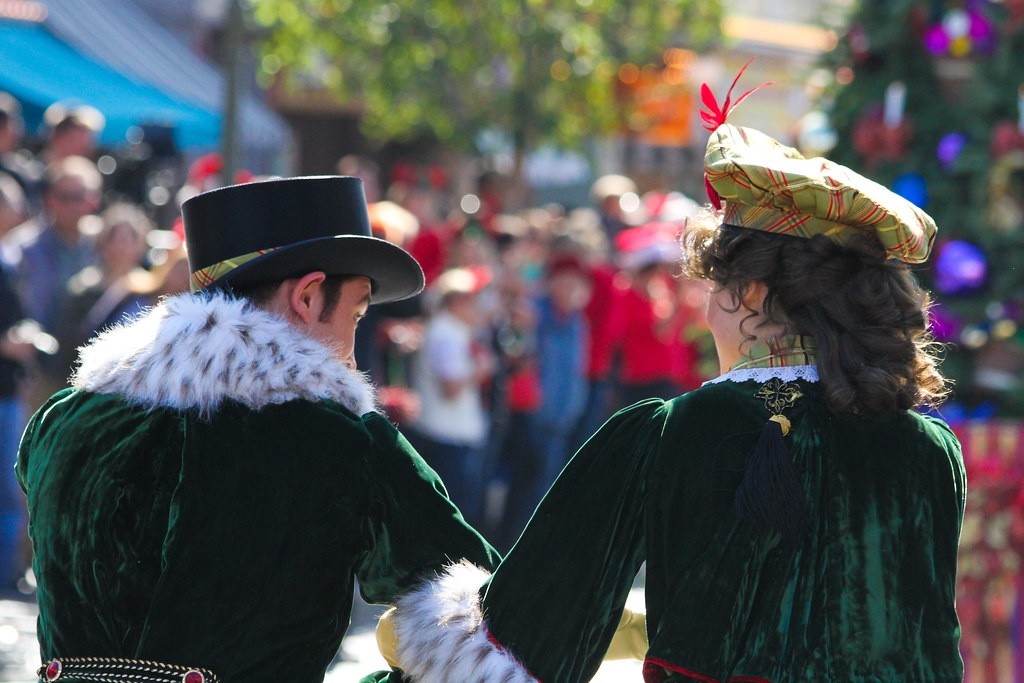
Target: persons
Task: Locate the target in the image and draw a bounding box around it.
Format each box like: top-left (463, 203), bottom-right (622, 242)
top-left (13, 175), bottom-right (649, 683)
top-left (374, 54), bottom-right (966, 683)
top-left (1, 91), bottom-right (721, 604)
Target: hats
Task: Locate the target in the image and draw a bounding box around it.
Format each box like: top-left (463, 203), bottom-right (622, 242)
top-left (181, 177), bottom-right (425, 306)
top-left (698, 56), bottom-right (937, 262)
